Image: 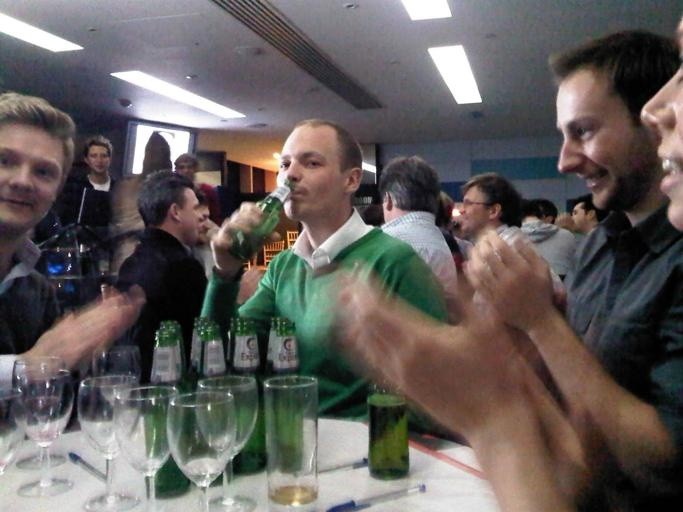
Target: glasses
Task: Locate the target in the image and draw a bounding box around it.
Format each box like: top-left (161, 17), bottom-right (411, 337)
top-left (460, 199), bottom-right (494, 211)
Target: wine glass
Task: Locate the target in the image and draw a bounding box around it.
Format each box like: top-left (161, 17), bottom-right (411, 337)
top-left (12, 368), bottom-right (75, 501)
top-left (167, 393), bottom-right (233, 510)
top-left (77, 375), bottom-right (139, 510)
top-left (113, 384), bottom-right (180, 510)
top-left (194, 375), bottom-right (259, 510)
top-left (13, 355), bottom-right (67, 472)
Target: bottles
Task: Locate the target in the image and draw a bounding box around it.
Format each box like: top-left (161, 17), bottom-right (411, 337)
top-left (226, 180), bottom-right (294, 262)
top-left (142, 315), bottom-right (300, 499)
top-left (368, 370), bottom-right (410, 480)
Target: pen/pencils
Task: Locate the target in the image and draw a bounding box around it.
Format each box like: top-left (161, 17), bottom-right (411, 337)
top-left (293, 457), bottom-right (368, 477)
top-left (327, 485), bottom-right (426, 512)
top-left (69, 451), bottom-right (107, 485)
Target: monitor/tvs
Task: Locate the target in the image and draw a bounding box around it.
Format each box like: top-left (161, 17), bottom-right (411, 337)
top-left (121, 117), bottom-right (197, 179)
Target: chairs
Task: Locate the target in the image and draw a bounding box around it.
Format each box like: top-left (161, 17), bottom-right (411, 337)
top-left (241, 230), bottom-right (299, 272)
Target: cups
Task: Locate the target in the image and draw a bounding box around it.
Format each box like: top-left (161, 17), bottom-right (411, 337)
top-left (263, 376), bottom-right (319, 505)
